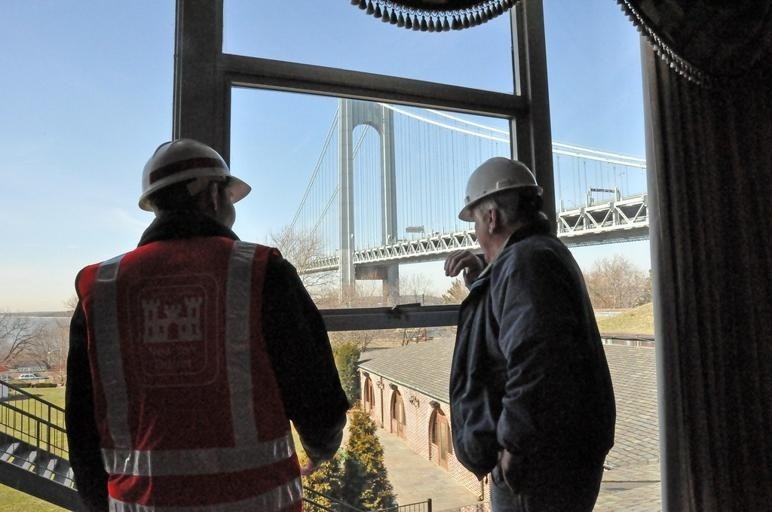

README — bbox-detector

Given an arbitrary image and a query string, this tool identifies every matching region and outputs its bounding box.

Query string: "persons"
[444,158,616,512]
[65,140,351,512]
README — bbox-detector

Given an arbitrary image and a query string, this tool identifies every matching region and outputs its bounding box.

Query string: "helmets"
[458,155,544,223]
[137,137,252,213]
